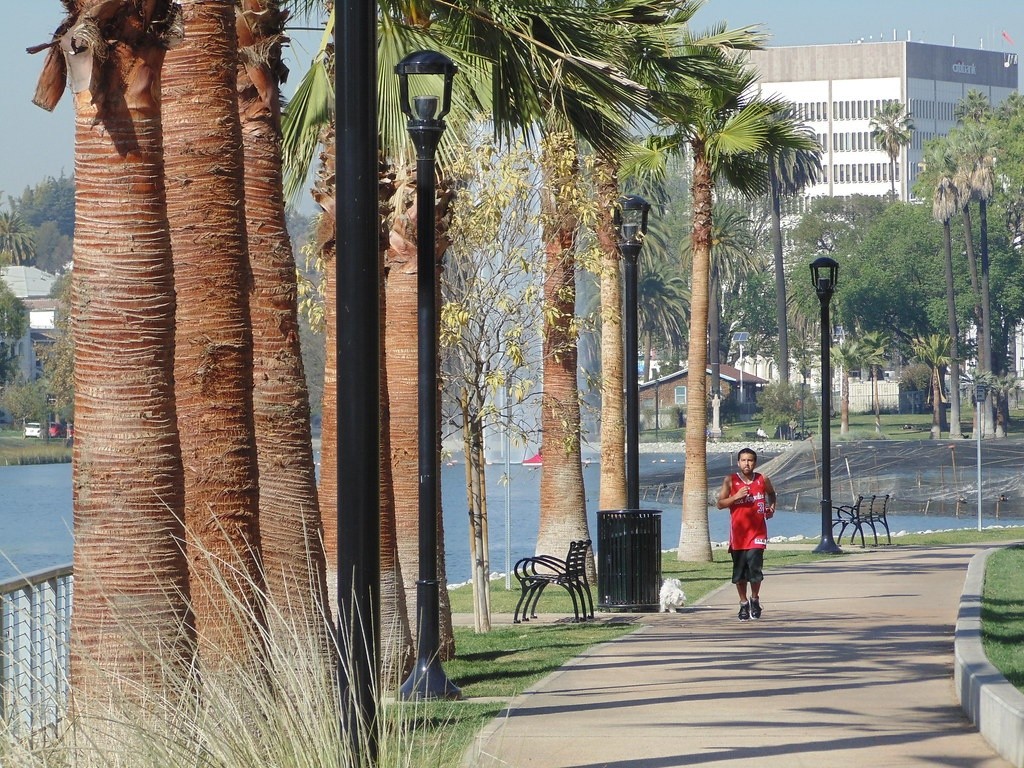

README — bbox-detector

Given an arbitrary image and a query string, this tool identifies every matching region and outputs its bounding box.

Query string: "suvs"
[25,423,41,439]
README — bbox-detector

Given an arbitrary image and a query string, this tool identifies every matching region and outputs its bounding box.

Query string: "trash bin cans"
[597,509,663,611]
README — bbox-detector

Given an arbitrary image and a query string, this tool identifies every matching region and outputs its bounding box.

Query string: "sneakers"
[737,598,750,622]
[750,596,764,619]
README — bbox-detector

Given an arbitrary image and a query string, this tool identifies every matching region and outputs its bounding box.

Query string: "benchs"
[746,432,769,442]
[514,539,594,624]
[831,495,891,548]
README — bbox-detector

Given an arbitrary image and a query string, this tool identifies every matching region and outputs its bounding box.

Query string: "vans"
[49,423,61,439]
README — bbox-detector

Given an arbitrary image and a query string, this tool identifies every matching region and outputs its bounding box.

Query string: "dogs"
[658,577,687,613]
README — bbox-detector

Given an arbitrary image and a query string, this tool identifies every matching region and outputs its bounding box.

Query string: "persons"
[717,448,776,621]
[757,427,769,441]
[789,418,798,440]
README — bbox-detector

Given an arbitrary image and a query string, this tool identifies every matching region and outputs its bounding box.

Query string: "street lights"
[808,258,844,555]
[396,48,461,705]
[610,194,653,512]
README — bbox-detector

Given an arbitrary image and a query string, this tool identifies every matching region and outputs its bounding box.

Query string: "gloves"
[765,507,774,520]
